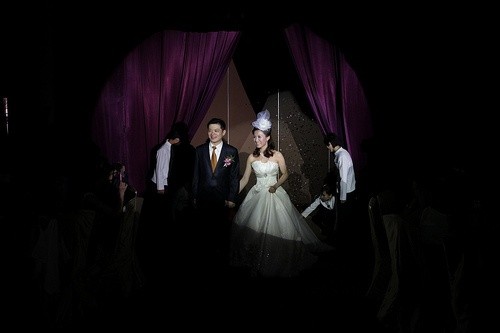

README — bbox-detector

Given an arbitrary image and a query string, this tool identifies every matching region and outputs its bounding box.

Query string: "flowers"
[223,152,235,167]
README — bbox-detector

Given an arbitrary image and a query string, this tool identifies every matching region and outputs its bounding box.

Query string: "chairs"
[368,191,471,333]
[41,196,144,333]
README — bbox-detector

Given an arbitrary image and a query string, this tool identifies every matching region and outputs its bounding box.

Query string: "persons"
[70,161,128,333]
[191,118,238,271]
[300,182,335,237]
[144,121,188,239]
[326,132,358,238]
[229,110,323,278]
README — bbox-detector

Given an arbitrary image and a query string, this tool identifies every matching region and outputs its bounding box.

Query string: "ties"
[211,146,218,172]
